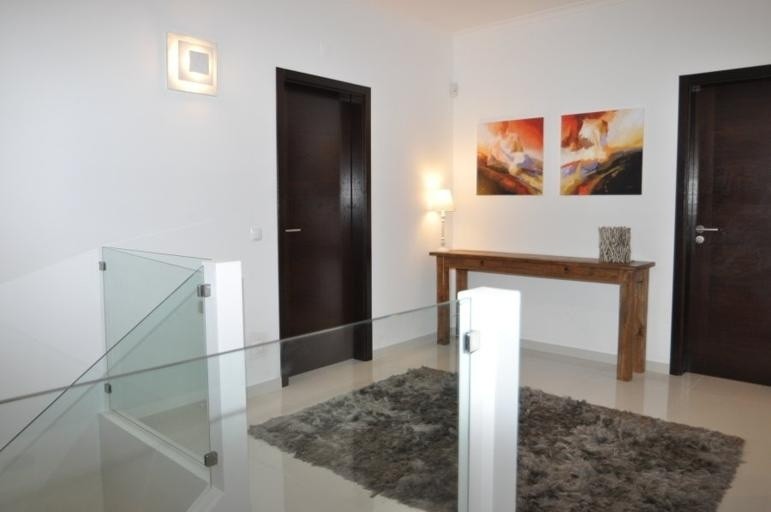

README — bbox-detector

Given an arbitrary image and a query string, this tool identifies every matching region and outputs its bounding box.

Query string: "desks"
[430,247,655,381]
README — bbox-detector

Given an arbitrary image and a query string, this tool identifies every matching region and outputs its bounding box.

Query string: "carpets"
[248,367,745,512]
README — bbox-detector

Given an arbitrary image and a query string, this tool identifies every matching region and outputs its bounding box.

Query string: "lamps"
[166,32,217,96]
[429,189,456,251]
